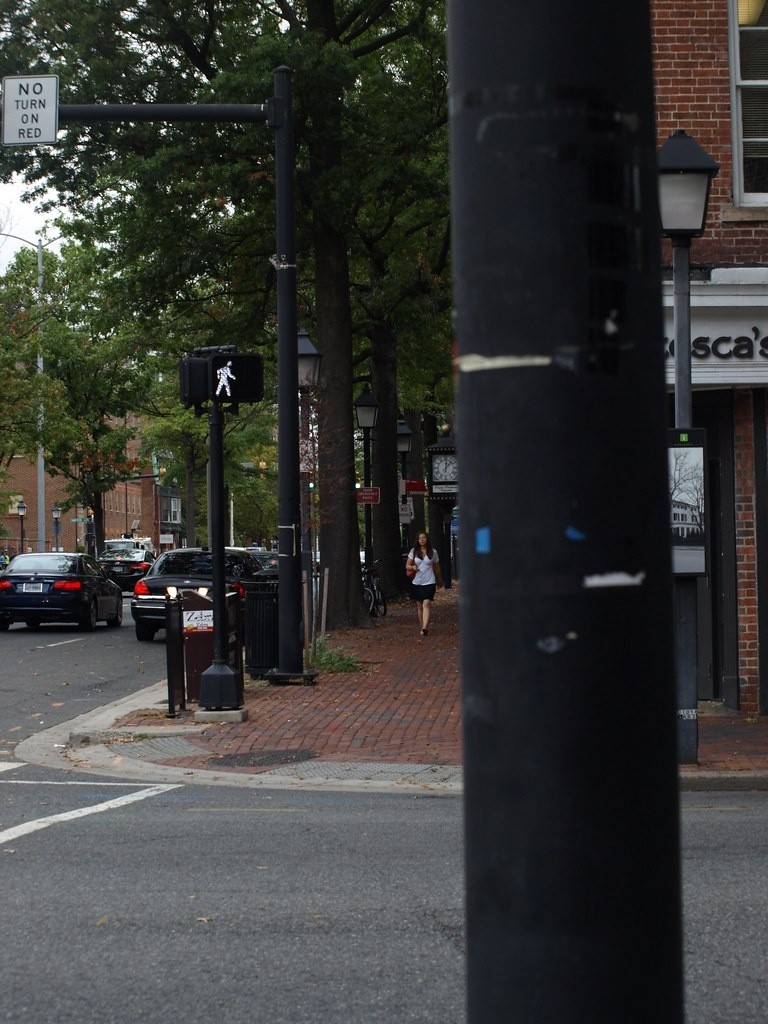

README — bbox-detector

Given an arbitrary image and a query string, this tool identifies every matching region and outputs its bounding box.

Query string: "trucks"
[104,537,153,555]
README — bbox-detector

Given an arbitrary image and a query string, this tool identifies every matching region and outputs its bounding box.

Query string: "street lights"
[51,506,62,552]
[398,421,413,555]
[656,129,718,767]
[353,382,382,615]
[17,501,27,554]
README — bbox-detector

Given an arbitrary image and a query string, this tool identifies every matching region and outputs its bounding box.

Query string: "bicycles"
[360,559,386,617]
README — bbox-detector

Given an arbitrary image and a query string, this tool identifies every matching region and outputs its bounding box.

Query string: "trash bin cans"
[182,587,245,703]
[240,575,306,682]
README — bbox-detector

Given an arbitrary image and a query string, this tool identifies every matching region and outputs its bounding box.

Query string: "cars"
[249,551,320,582]
[0,552,124,633]
[132,545,268,642]
[97,549,157,592]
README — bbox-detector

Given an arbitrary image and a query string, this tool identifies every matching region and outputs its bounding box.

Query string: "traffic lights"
[206,352,265,403]
[179,355,209,410]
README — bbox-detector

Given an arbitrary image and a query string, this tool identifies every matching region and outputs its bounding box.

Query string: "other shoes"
[420,629,428,635]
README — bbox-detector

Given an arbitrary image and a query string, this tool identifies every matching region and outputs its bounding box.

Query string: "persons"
[0,550,10,570]
[405,533,445,637]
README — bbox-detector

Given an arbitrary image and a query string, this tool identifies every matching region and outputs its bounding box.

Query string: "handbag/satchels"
[407,547,417,578]
